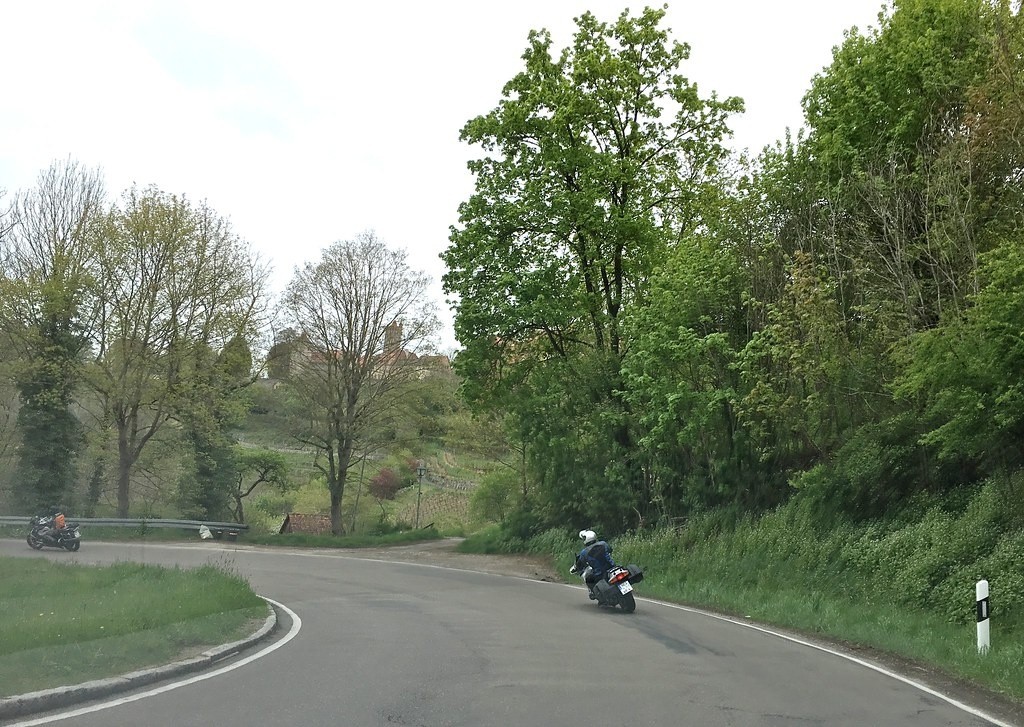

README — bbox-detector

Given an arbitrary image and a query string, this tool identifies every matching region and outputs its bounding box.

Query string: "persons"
[574,530,615,600]
[39,506,65,546]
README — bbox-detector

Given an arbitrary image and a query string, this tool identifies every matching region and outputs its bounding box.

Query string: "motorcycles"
[26,512,82,551]
[569,551,644,614]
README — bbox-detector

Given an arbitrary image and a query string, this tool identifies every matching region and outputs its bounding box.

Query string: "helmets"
[579,529,597,546]
